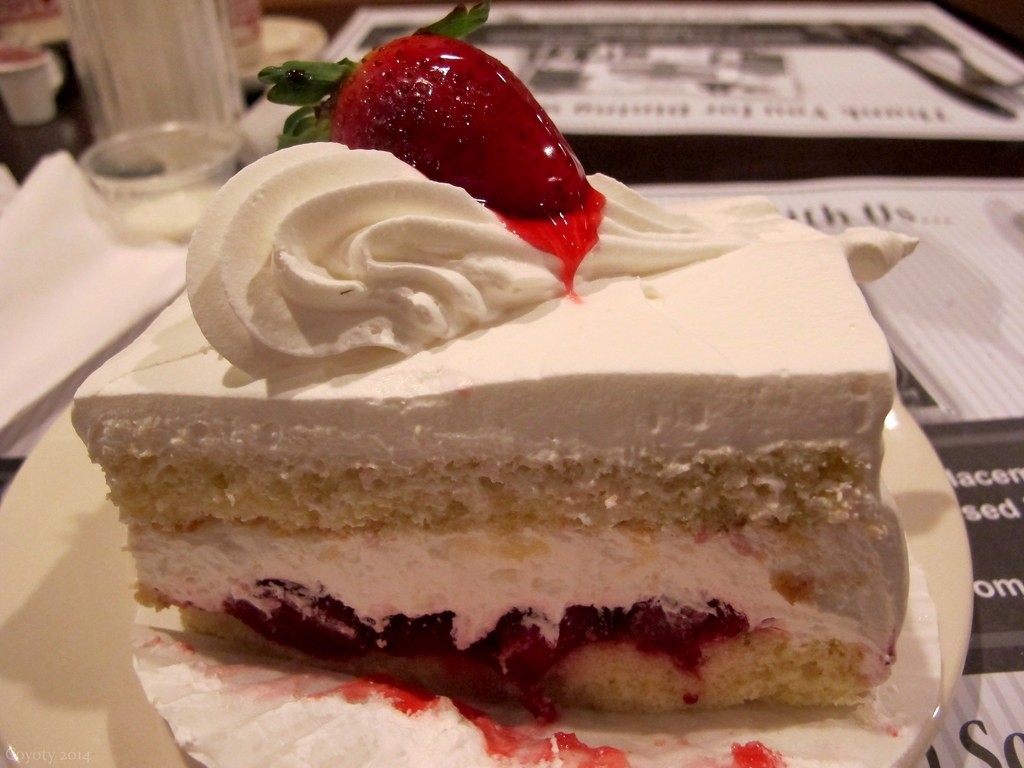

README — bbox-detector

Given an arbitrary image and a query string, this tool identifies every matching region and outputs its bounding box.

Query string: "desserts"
[65,1,922,724]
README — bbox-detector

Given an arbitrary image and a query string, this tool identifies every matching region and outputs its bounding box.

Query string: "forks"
[915,21,1024,117]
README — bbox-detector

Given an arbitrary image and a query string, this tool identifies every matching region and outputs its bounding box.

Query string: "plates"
[236,17,328,91]
[0,379,976,768]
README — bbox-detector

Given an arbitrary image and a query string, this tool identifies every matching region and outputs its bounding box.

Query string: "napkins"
[0,148,194,459]
[858,194,1024,422]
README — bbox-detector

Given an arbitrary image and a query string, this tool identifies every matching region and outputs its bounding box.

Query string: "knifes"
[849,22,1015,118]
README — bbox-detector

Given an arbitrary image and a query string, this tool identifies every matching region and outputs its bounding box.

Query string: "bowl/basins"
[78,121,245,245]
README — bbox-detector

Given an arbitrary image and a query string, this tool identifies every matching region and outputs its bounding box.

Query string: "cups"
[63,0,248,168]
[0,46,60,126]
